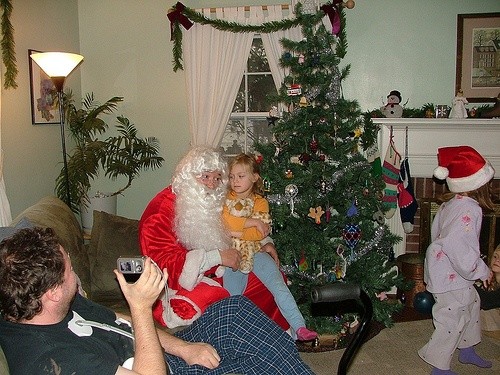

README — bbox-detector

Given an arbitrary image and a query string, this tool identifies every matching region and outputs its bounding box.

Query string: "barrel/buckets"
[395,253,426,309]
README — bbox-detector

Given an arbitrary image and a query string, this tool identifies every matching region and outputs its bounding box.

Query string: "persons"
[138,145,298,343]
[472,242,500,332]
[416,145,496,375]
[0,226,315,375]
[221,153,318,343]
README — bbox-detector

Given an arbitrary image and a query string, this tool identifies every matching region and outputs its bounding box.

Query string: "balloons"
[413,292,434,312]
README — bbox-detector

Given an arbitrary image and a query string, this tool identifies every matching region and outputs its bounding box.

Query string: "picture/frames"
[29,48,65,125]
[455,12,500,103]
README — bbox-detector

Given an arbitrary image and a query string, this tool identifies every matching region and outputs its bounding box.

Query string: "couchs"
[0,198,192,375]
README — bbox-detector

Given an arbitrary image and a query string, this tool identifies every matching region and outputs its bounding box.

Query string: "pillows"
[86,210,140,307]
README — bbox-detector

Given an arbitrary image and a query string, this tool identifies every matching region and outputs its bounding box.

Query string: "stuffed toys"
[449,89,469,118]
[382,89,403,118]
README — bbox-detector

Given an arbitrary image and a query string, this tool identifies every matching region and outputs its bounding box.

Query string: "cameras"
[116,255,145,274]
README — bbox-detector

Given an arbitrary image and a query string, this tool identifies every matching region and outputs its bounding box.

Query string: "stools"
[397,253,426,308]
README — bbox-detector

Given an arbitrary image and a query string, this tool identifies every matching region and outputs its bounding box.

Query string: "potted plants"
[54,87,165,235]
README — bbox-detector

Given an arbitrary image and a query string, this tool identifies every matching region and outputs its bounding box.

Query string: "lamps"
[30,52,84,211]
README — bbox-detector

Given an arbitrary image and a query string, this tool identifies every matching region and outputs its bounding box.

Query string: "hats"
[433,146,495,192]
